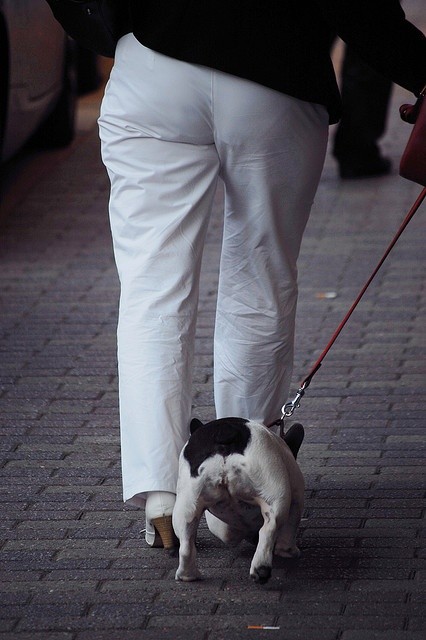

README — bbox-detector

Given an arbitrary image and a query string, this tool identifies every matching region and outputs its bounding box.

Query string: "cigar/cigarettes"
[316,292,337,299]
[247,626,280,630]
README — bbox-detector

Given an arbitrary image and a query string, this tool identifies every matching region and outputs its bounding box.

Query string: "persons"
[95,2,425,555]
[330,43,393,180]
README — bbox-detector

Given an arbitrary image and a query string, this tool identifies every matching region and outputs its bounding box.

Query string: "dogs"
[172,418,305,584]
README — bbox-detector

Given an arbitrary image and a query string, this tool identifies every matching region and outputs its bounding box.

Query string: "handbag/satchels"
[398,87,426,187]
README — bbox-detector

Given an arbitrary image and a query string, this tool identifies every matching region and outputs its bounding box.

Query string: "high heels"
[145,491,176,550]
